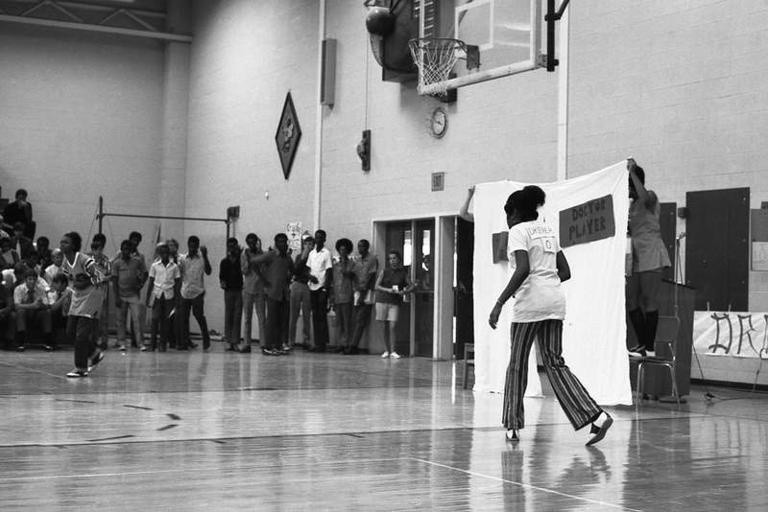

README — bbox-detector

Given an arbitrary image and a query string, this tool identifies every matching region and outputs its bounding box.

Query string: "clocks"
[431,107,448,138]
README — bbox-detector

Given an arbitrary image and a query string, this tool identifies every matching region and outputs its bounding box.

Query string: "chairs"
[629,316,680,409]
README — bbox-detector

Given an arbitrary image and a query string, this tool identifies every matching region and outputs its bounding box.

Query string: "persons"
[1,189,211,376]
[220,229,413,359]
[487,188,611,447]
[627,157,671,359]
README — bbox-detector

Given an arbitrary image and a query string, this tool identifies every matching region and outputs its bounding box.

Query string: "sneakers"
[507,430,519,442]
[382,352,388,357]
[203,342,210,351]
[88,352,104,373]
[263,344,292,356]
[312,344,356,355]
[225,343,251,352]
[629,345,656,357]
[45,345,53,350]
[391,352,400,359]
[67,368,88,377]
[131,340,197,353]
[119,345,127,351]
[585,412,612,446]
[16,346,24,352]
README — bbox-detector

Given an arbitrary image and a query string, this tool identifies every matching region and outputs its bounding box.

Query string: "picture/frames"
[275,92,301,179]
